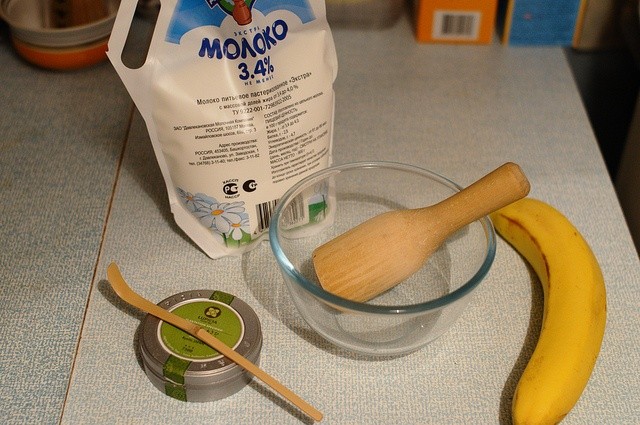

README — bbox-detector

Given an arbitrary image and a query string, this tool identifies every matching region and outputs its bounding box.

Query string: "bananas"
[488,197,608,425]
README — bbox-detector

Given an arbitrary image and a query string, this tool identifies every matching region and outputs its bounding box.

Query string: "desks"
[0,28,640,424]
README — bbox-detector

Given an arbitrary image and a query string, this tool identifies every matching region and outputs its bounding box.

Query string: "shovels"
[312,162,530,312]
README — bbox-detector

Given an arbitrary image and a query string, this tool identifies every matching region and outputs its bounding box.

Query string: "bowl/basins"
[266,160,499,355]
[0,0,121,74]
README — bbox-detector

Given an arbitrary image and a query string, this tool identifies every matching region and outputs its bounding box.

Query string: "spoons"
[107,262,330,422]
